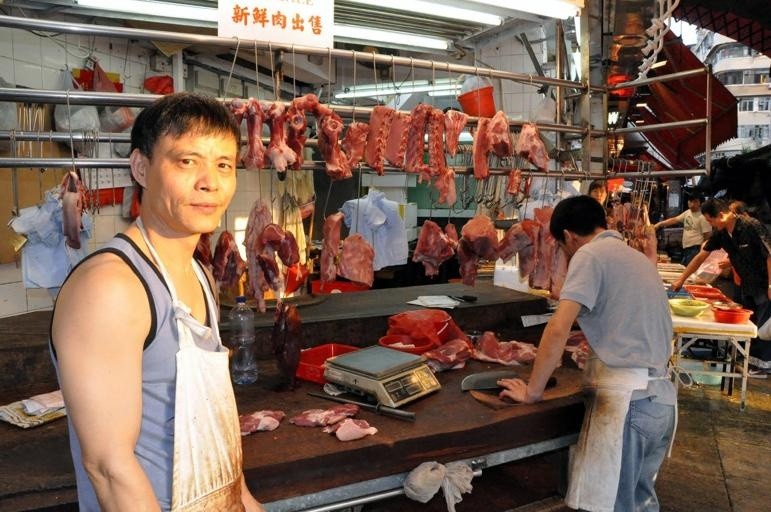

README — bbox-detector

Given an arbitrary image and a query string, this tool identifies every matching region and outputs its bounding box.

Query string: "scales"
[323,345,442,408]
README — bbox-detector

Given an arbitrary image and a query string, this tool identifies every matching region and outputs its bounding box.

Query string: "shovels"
[520,314,554,327]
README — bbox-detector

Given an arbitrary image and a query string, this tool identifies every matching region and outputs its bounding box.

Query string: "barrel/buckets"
[454,73,497,119]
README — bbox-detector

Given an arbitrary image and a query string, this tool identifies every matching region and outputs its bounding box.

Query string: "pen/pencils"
[448,295,465,302]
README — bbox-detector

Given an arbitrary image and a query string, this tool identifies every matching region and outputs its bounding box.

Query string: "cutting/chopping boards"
[470,364,582,410]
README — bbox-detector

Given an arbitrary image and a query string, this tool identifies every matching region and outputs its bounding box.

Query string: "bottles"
[228,296,258,385]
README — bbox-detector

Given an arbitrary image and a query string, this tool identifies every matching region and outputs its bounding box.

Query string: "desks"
[665,281,759,415]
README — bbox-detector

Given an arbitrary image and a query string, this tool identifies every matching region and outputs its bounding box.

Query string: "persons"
[587,179,607,204]
[496,197,678,512]
[48,93,269,512]
[651,192,771,379]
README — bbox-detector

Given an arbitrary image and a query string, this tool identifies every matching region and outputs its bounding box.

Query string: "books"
[419,293,460,307]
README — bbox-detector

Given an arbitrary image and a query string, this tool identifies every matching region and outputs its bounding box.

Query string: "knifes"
[460,369,558,391]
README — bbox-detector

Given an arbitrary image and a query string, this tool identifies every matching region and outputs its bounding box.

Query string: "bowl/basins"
[668,298,710,317]
[711,307,754,324]
[688,361,736,387]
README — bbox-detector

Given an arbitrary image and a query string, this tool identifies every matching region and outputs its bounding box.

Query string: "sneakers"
[737,364,768,379]
